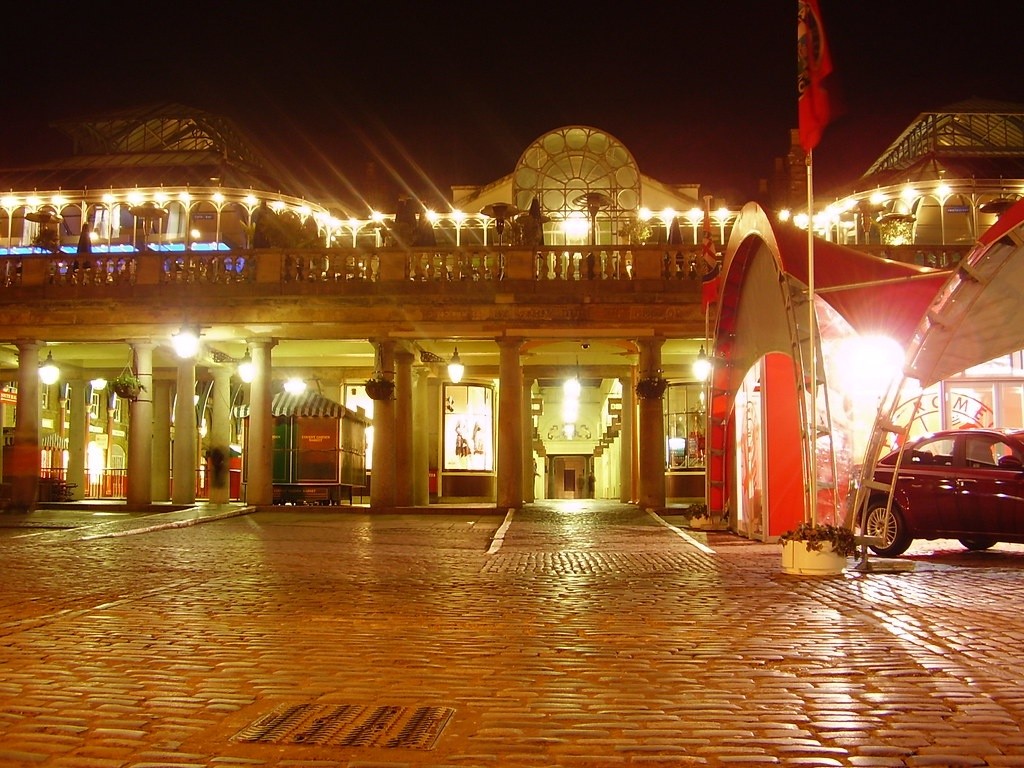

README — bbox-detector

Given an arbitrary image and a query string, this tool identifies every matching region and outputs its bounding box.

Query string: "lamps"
[238,347,257,383]
[37,350,60,385]
[447,346,464,385]
[90,377,108,391]
[693,342,712,381]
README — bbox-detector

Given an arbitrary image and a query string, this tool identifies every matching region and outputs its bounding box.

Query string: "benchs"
[910,451,955,466]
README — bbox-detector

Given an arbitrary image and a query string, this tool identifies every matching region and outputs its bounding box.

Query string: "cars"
[853,429,1024,557]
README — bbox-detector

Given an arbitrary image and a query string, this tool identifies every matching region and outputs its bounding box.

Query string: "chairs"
[969,439,995,468]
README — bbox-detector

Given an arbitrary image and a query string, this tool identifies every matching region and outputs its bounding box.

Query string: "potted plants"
[635,376,670,399]
[111,375,148,398]
[779,519,856,575]
[683,503,728,530]
[364,371,396,400]
